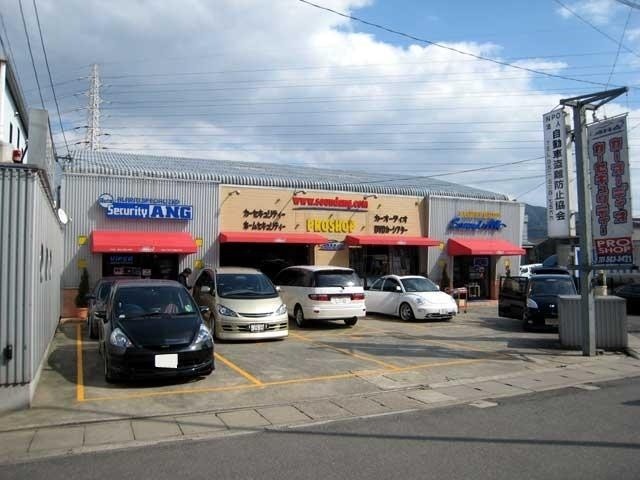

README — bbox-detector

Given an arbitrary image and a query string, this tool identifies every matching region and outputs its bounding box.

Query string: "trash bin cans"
[557,295,627,350]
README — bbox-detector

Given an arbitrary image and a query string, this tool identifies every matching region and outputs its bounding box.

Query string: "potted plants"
[75,268,89,320]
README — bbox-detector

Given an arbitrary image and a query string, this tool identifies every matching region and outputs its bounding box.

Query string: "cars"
[193,267,289,340]
[272,265,367,328]
[498,254,578,327]
[85,275,214,384]
[365,274,458,322]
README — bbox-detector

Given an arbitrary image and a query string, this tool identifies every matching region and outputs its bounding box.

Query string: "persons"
[176,267,193,291]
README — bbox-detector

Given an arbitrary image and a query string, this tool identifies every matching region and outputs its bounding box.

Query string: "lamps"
[294,190,307,195]
[229,190,240,195]
[364,194,378,199]
